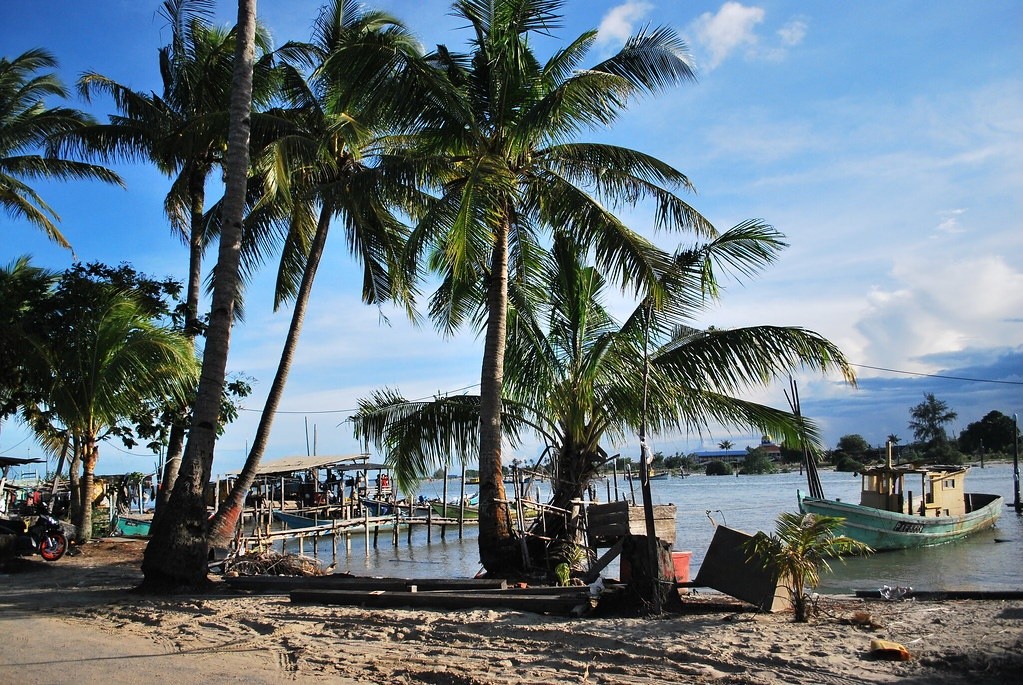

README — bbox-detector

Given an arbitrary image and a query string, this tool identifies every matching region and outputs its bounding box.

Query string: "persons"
[373,474,388,501]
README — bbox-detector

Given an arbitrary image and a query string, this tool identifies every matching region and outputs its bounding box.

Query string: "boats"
[781,375,1005,558]
[94,451,553,546]
[624,468,672,481]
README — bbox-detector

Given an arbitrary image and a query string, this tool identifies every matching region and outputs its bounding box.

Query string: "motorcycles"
[0,499,69,561]
